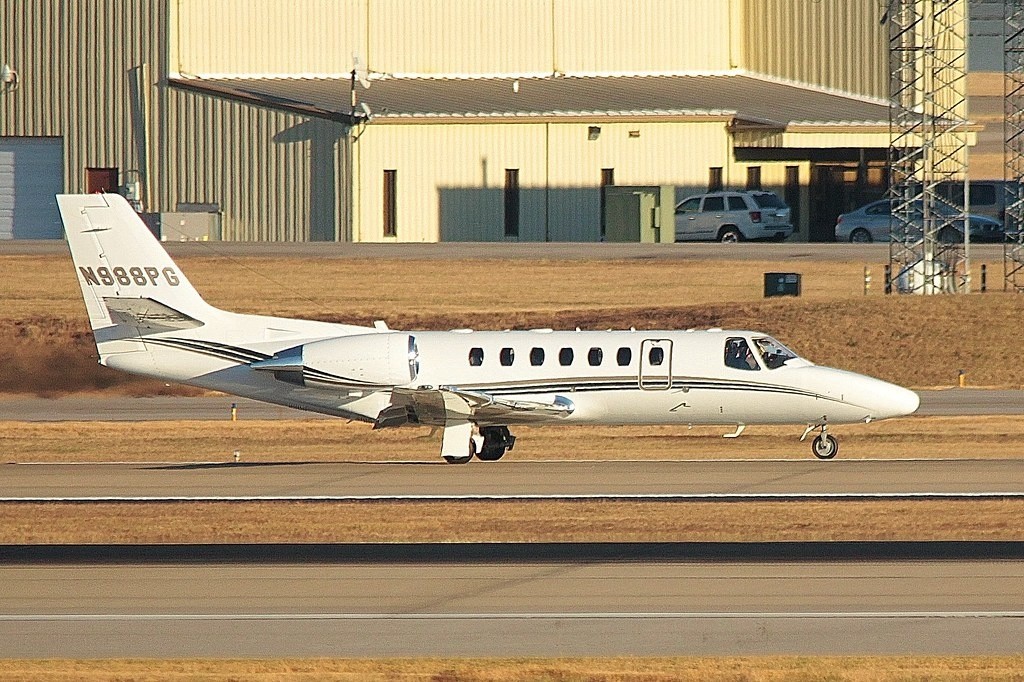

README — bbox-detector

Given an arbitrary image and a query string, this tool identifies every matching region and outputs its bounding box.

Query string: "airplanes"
[56,193,921,464]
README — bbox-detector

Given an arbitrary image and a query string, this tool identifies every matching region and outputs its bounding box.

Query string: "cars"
[835,198,1005,245]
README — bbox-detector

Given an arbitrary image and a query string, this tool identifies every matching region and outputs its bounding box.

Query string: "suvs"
[674,190,792,243]
